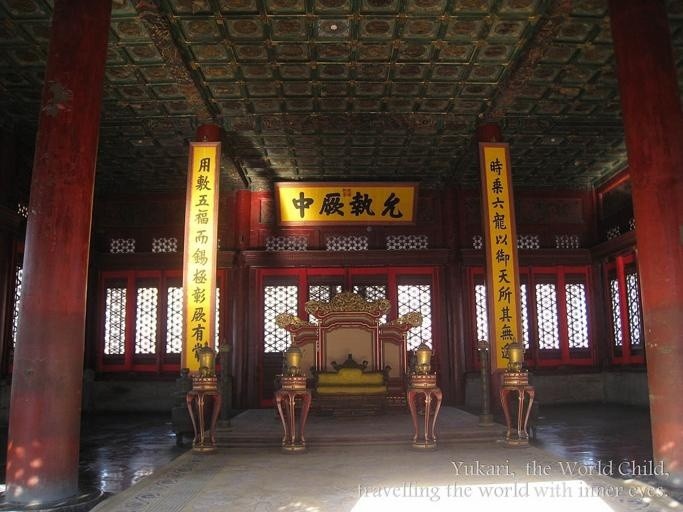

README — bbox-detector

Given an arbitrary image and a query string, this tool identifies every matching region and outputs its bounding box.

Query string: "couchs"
[313,353,393,397]
